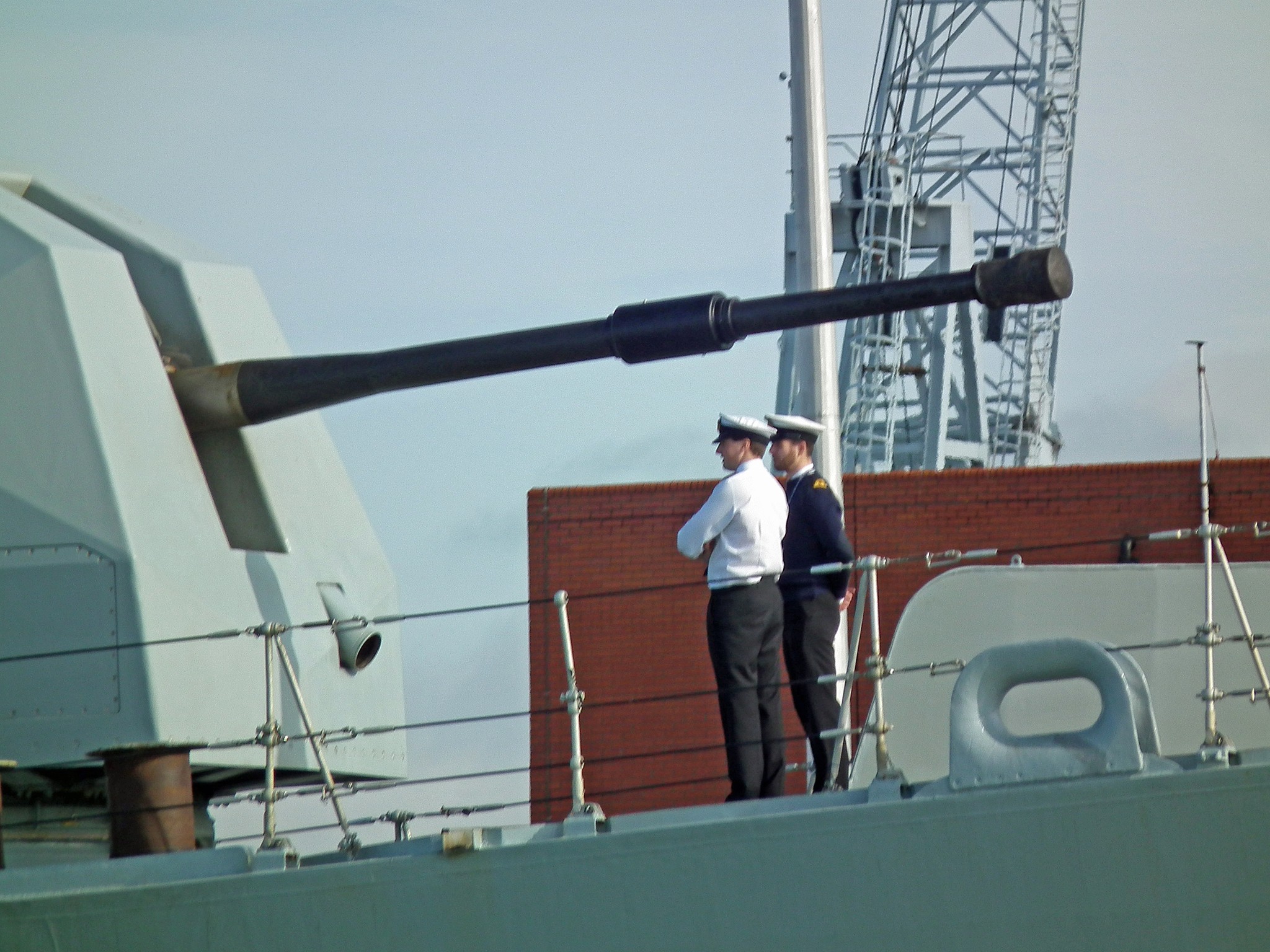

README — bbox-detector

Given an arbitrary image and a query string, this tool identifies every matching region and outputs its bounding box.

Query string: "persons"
[764,413,856,794]
[677,411,788,803]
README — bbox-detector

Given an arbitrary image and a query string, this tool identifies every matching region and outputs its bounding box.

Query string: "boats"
[3,0,1269,952]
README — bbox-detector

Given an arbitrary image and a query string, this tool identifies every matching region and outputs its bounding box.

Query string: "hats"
[712,412,777,443]
[764,415,826,443]
